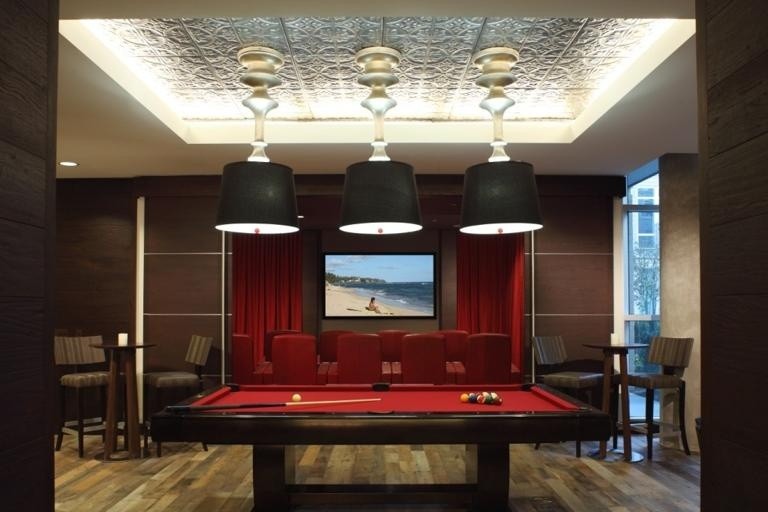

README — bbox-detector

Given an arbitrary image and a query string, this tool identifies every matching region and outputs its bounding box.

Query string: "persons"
[368,297,381,313]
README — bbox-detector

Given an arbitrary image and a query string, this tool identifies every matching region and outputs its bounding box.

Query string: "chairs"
[52,334,126,457]
[231,328,520,385]
[531,335,604,458]
[611,336,694,460]
[143,334,215,456]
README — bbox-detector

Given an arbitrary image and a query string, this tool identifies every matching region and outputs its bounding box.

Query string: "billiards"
[291,394,302,402]
[462,392,503,405]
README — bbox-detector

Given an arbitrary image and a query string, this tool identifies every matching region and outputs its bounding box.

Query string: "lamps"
[214,44,301,237]
[459,46,545,237]
[338,45,424,237]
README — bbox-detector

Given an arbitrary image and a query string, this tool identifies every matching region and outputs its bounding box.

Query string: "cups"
[118,333,129,347]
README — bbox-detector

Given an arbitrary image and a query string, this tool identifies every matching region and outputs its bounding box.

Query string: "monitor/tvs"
[321,251,438,321]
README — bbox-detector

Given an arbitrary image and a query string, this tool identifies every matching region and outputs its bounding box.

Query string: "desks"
[88,342,158,462]
[150,382,613,512]
[580,342,650,464]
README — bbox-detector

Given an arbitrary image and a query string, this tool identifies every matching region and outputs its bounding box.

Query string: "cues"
[166,398,382,410]
